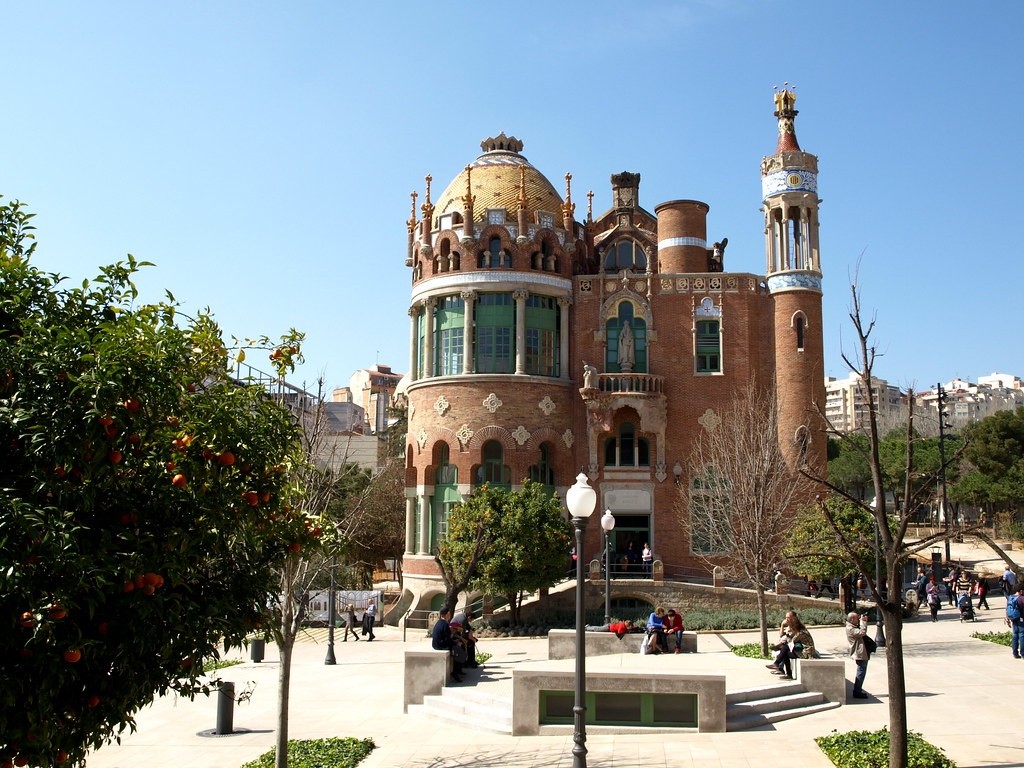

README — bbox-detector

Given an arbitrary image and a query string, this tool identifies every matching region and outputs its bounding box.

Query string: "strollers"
[956,592,976,623]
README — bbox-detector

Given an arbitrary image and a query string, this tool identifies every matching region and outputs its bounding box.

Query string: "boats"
[320,521,342,666]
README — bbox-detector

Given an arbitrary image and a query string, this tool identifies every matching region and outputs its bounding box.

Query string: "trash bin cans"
[251,638,264,663]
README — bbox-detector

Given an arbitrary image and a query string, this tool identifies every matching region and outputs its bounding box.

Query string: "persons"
[926,576,941,622]
[603,545,617,580]
[568,541,576,580]
[945,566,990,610]
[1006,584,1024,658]
[617,320,635,364]
[450,610,480,668]
[342,604,360,642]
[583,365,598,388]
[646,608,684,654]
[642,543,652,578]
[846,612,868,699]
[364,599,376,642]
[625,542,633,576]
[585,620,641,634]
[914,567,928,607]
[1004,566,1016,598]
[766,611,821,679]
[802,569,836,601]
[432,607,466,682]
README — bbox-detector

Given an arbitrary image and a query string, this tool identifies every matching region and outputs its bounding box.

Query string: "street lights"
[566,468,597,768]
[601,507,615,627]
[869,496,886,648]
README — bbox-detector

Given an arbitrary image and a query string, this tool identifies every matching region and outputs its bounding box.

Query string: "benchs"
[772,646,845,704]
[513,660,726,735]
[403,643,459,714]
[548,629,697,662]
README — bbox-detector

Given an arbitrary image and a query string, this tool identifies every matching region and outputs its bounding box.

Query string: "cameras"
[862,612,868,617]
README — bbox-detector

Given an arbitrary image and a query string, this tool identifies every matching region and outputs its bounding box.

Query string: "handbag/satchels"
[640,635,652,654]
[863,635,877,653]
[978,587,984,596]
[928,594,936,604]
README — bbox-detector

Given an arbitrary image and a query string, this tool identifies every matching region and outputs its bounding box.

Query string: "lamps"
[670,458,684,487]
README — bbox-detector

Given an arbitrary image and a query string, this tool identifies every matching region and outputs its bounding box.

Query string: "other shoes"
[976,606,980,609]
[766,663,778,669]
[780,674,793,680]
[772,670,785,674]
[342,639,348,641]
[1013,654,1022,659]
[675,648,679,653]
[661,648,667,653]
[355,637,359,640]
[367,634,375,642]
[451,671,463,683]
[853,692,868,698]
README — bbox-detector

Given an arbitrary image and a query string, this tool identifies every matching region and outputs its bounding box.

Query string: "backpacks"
[1007,595,1020,620]
